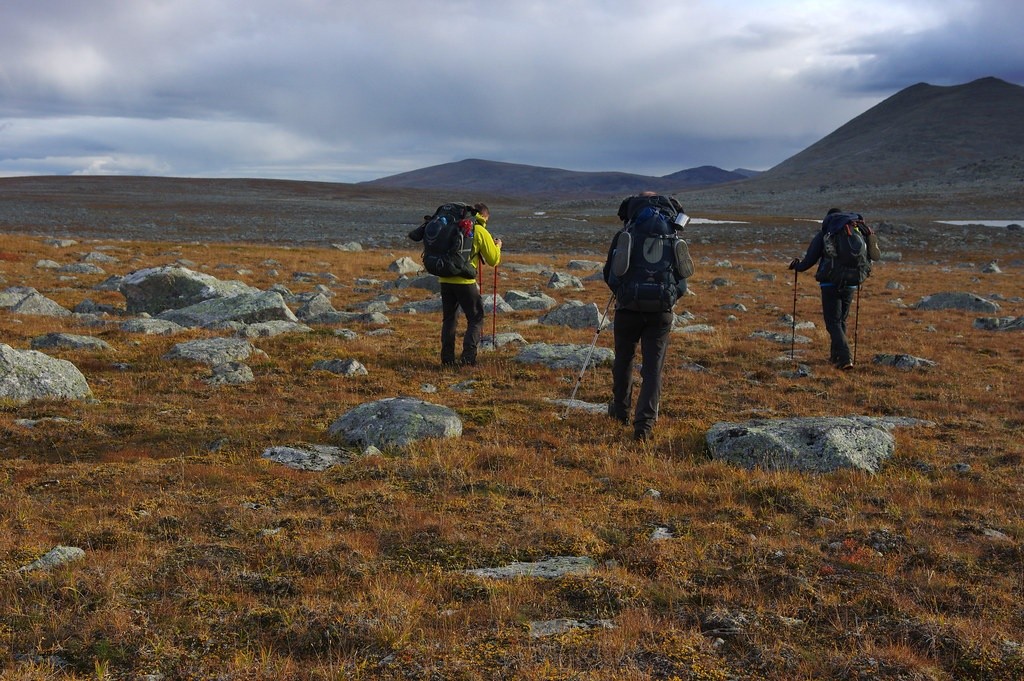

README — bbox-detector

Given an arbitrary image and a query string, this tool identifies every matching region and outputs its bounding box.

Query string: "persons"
[789,208,856,370]
[437,202,502,372]
[602,192,687,444]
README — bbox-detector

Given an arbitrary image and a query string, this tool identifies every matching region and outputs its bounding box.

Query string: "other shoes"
[441,361,459,371]
[867,235,881,260]
[634,428,646,441]
[824,234,835,255]
[829,357,837,365]
[675,239,696,278]
[608,406,630,426]
[611,232,630,276]
[837,358,853,370]
[462,357,480,367]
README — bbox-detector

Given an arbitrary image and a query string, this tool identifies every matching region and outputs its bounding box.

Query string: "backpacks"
[827,219,872,284]
[422,203,478,278]
[609,194,691,281]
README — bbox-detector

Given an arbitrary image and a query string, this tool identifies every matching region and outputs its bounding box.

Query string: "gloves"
[789,259,801,270]
